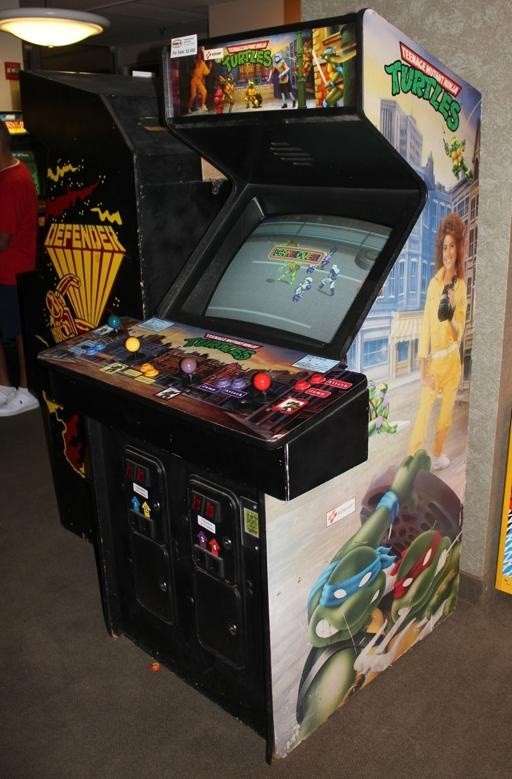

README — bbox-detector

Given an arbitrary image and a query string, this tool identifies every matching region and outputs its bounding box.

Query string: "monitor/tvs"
[204,213,393,344]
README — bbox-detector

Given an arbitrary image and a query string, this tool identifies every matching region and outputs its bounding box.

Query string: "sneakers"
[430,453,450,470]
[0,385,40,417]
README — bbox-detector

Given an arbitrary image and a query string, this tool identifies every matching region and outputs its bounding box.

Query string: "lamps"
[1,8,110,50]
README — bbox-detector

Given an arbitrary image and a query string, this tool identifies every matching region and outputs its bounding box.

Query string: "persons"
[0,117,49,418]
[406,211,469,476]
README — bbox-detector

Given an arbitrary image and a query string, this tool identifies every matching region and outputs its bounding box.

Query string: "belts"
[432,344,457,360]
